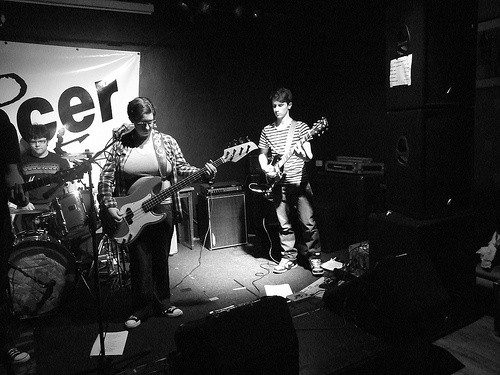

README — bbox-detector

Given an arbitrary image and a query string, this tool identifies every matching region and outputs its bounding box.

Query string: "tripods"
[100,246,132,285]
[43,138,152,375]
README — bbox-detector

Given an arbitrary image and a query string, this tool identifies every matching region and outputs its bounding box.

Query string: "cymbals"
[9,207,42,214]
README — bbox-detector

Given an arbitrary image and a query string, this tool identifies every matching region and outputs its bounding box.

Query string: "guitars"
[101,135,258,246]
[0,164,85,205]
[259,116,329,208]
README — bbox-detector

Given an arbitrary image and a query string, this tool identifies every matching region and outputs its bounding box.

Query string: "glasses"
[136,119,156,127]
[30,139,48,145]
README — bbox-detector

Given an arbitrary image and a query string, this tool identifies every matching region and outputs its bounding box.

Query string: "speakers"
[383,112,473,216]
[310,171,371,251]
[326,251,416,335]
[365,210,477,342]
[172,295,300,375]
[196,191,249,250]
[383,0,476,111]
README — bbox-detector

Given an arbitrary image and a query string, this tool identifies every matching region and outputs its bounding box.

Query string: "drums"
[53,190,88,233]
[5,229,80,321]
[36,210,67,240]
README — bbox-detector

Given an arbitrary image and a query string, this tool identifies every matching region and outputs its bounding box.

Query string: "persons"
[258,88,324,276]
[97,97,217,327]
[0,110,30,362]
[13,125,92,239]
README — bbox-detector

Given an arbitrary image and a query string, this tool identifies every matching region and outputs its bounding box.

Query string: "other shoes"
[273,258,298,273]
[309,258,325,274]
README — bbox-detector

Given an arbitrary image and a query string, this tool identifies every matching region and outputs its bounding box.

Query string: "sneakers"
[2,348,30,364]
[159,305,183,317]
[124,310,146,329]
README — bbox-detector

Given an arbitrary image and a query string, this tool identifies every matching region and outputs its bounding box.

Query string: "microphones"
[47,280,56,287]
[114,122,131,138]
